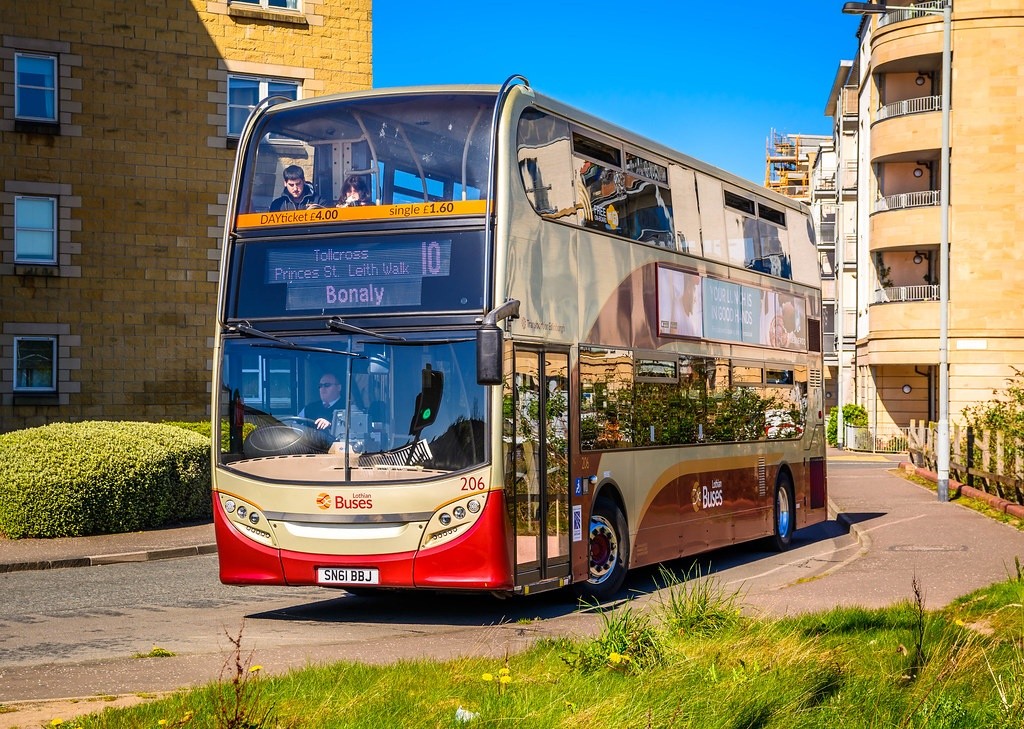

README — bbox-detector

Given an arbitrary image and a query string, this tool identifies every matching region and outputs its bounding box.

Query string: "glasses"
[317,383,337,388]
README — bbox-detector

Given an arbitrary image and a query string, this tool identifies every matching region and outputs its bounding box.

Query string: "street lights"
[841,1,954,503]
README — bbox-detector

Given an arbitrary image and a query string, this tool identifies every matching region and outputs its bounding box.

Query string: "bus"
[209,73,831,610]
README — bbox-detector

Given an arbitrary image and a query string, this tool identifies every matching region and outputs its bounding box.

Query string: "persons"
[334,174,377,208]
[270,164,334,212]
[293,371,365,430]
